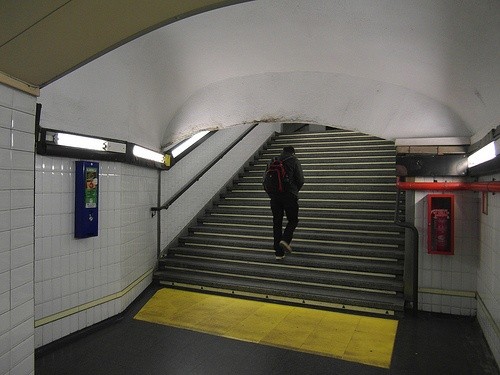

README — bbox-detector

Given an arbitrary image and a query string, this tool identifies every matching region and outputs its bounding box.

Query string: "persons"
[264,146,305,259]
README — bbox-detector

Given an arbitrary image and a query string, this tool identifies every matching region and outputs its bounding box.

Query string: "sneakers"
[279,240,291,254]
[276,255,286,259]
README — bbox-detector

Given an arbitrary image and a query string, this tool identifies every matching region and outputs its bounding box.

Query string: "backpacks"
[262,156,295,197]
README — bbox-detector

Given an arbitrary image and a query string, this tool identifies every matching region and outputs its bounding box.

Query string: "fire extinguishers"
[435,210,447,250]
